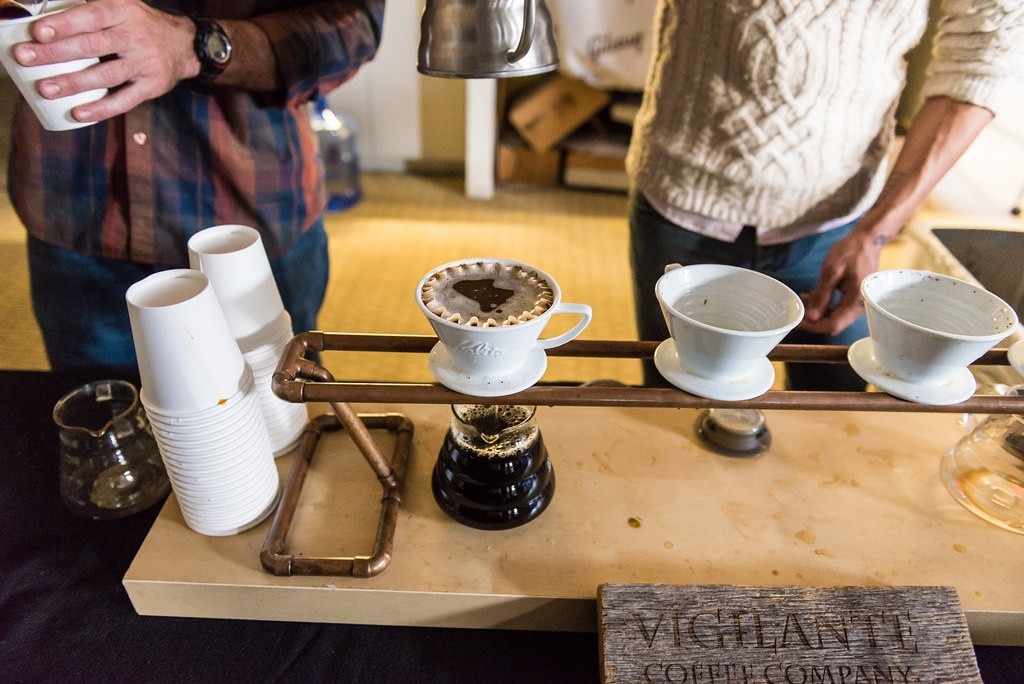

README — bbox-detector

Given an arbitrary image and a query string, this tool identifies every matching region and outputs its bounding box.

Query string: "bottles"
[307,97,361,213]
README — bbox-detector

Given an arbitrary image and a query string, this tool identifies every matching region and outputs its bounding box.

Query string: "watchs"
[183,10,233,92]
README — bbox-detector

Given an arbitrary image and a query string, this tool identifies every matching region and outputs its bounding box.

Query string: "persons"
[0,0,384,377]
[625,0,1024,393]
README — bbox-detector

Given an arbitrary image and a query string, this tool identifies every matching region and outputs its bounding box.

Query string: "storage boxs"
[499,145,560,189]
[510,74,609,152]
[565,142,631,193]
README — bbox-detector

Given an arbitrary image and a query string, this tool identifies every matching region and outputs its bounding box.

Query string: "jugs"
[430,402,555,531]
[51,376,171,520]
[941,382,1024,532]
[417,0,560,79]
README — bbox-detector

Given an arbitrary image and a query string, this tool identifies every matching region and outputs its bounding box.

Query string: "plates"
[1006,340,1024,381]
[848,336,977,406]
[428,338,549,398]
[654,337,775,402]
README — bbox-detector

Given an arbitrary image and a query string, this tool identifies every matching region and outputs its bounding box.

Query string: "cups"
[125,223,310,538]
[656,262,805,381]
[414,256,594,380]
[861,270,1017,384]
[0,0,110,131]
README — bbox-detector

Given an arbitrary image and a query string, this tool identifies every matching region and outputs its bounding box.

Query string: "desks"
[0,368,1024,684]
